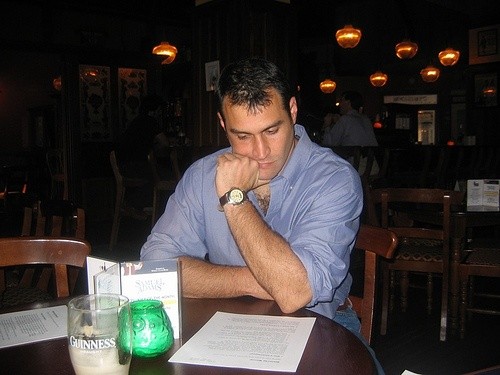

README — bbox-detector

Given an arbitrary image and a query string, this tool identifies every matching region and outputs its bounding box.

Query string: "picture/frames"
[204,58,221,95]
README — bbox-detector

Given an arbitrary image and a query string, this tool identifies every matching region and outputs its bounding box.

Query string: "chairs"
[0,200,92,304]
[170,145,184,178]
[110,150,159,250]
[339,180,500,345]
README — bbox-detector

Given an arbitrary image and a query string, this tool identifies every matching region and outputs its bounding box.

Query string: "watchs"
[219,187,249,212]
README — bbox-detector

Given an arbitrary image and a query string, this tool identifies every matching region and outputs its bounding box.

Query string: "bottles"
[374,112,385,146]
[119,299,173,358]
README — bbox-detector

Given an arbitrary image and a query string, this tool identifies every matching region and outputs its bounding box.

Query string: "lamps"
[152,30,180,65]
[319,14,461,94]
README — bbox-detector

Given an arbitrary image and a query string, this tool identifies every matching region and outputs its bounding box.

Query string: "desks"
[0,296,377,375]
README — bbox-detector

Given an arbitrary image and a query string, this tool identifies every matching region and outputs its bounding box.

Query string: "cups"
[66,293,132,375]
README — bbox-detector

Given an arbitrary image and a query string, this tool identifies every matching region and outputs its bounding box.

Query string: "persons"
[121,95,181,211]
[322,91,379,187]
[139,59,385,375]
